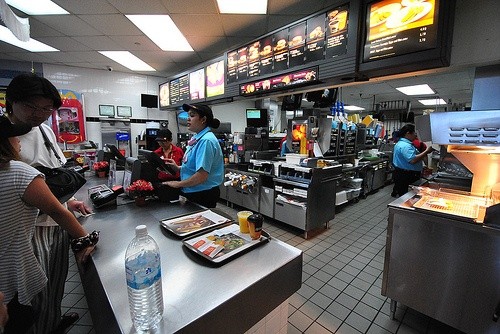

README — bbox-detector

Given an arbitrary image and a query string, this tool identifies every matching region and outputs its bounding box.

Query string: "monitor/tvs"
[106,144,126,161]
[138,149,177,175]
[141,94,158,108]
[246,109,267,127]
[89,140,98,149]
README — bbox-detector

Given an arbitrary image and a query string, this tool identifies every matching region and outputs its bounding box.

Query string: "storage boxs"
[286,154,309,165]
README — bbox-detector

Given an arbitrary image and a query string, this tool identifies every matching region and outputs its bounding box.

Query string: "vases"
[97,171,106,178]
[133,193,145,206]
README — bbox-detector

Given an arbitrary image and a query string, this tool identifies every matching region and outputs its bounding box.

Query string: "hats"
[405,124,415,132]
[154,129,172,141]
[183,103,213,122]
[0,110,32,137]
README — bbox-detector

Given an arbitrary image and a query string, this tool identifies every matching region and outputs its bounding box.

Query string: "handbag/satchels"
[33,125,87,216]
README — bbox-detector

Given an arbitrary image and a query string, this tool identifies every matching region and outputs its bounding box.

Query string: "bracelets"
[71,230,100,252]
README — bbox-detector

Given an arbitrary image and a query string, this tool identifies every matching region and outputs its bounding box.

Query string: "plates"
[370,2,401,28]
[385,2,433,29]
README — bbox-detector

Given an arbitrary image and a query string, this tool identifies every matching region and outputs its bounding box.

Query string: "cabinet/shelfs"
[219,164,342,238]
[320,122,358,164]
[358,129,383,152]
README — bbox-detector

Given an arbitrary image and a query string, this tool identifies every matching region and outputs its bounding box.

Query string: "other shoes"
[59,312,79,332]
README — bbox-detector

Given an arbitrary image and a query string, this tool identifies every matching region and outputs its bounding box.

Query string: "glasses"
[20,100,54,112]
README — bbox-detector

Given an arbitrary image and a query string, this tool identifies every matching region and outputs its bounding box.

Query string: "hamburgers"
[293,36,302,43]
[277,39,286,50]
[264,45,272,54]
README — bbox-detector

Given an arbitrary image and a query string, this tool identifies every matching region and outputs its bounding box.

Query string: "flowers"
[126,179,154,194]
[92,160,108,171]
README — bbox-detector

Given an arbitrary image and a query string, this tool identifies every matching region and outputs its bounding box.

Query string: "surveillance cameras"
[107,67,113,72]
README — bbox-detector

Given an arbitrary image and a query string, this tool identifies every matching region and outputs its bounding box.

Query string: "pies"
[310,26,322,38]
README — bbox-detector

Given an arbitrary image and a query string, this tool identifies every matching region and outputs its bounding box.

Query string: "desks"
[63,160,304,334]
[382,189,500,334]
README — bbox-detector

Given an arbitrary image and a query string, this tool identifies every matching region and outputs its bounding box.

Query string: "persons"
[0,71,93,334]
[162,103,224,208]
[153,129,184,195]
[389,124,433,198]
[0,113,94,334]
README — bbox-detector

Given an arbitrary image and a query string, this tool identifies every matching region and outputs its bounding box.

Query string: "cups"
[246,213,264,240]
[328,9,348,32]
[237,210,254,234]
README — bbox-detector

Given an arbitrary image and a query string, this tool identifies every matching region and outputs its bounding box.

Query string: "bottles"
[124,224,164,330]
